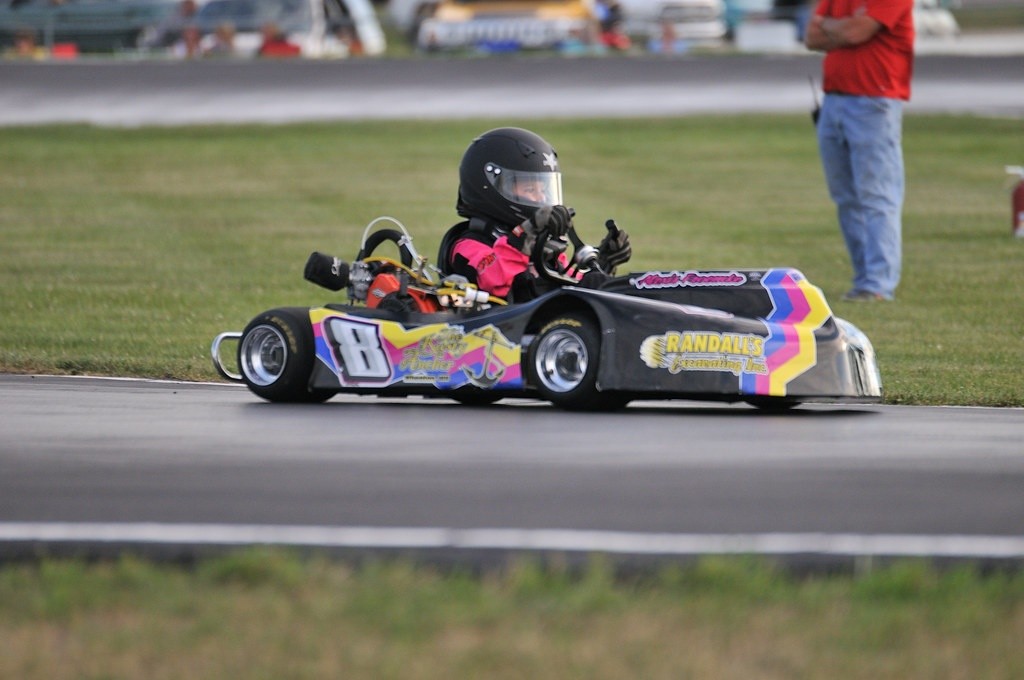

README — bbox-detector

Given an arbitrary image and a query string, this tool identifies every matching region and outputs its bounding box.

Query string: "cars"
[0,0,385,61]
[411,1,809,59]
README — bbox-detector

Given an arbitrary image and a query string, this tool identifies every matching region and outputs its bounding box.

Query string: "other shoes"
[844,286,884,302]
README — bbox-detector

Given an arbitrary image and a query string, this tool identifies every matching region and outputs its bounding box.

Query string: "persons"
[599,5,633,51]
[178,22,371,61]
[2,24,81,61]
[432,126,634,307]
[803,0,915,302]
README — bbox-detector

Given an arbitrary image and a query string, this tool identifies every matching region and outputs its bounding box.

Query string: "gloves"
[521,205,571,240]
[597,230,632,272]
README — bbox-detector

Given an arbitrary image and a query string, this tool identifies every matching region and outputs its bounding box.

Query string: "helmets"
[456,127,563,227]
